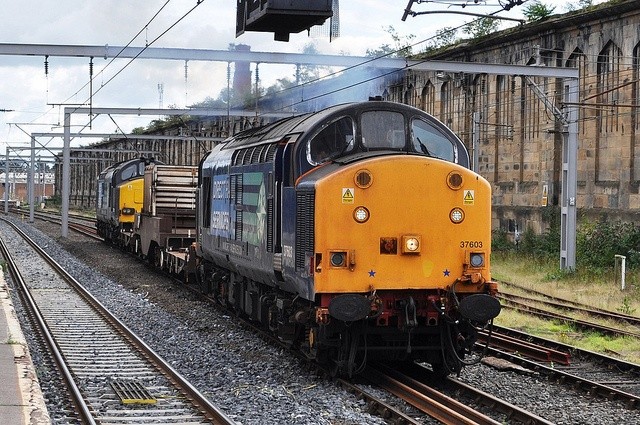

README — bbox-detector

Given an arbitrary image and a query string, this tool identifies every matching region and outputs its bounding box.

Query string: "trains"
[95,96,500,383]
